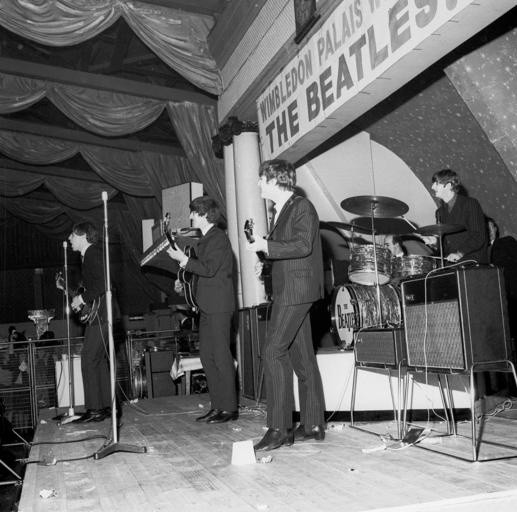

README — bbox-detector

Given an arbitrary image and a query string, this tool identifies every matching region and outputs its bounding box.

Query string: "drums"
[390,255,435,282]
[347,244,393,287]
[328,283,402,350]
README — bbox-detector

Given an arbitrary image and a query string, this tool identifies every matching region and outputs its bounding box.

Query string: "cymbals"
[414,224,466,236]
[341,196,408,219]
[351,217,418,234]
[328,222,378,235]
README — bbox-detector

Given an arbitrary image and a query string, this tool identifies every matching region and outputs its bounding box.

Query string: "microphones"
[101,191,108,227]
[62,241,68,265]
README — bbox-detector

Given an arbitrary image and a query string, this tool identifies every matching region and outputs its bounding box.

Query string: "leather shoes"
[205,409,239,422]
[70,408,109,424]
[195,407,221,422]
[72,405,123,417]
[292,423,325,441]
[253,424,294,451]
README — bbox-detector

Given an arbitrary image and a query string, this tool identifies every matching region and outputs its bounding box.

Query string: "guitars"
[244,219,273,301]
[54,272,100,325]
[163,212,200,315]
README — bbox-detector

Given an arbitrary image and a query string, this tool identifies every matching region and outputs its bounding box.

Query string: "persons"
[68,219,122,424]
[174,309,191,356]
[428,169,490,270]
[241,159,327,453]
[174,195,239,424]
[8,325,58,407]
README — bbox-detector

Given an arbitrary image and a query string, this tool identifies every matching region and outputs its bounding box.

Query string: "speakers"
[399,263,512,374]
[352,328,407,369]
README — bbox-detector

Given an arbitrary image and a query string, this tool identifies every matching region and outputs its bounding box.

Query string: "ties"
[443,202,451,218]
